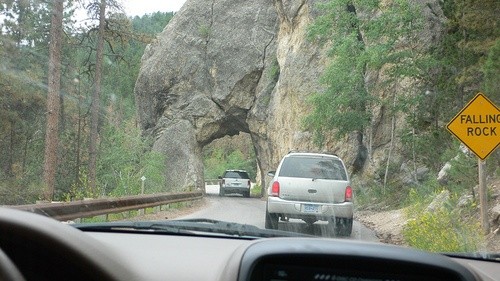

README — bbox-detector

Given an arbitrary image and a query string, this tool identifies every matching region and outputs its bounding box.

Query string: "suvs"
[218,168,252,196]
[265,151,353,236]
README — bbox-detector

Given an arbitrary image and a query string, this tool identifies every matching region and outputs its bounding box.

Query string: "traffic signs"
[447,93,500,158]
